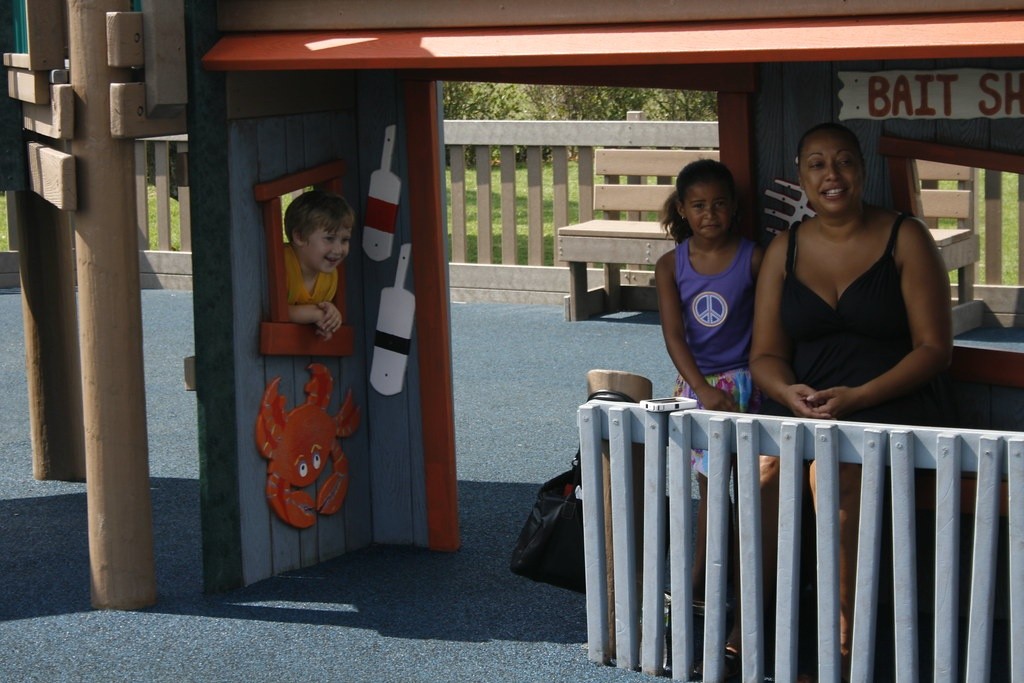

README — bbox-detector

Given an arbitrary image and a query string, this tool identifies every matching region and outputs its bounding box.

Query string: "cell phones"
[639,396,697,411]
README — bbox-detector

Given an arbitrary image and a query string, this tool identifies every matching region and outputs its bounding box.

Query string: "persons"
[654,121,953,683]
[284,190,357,342]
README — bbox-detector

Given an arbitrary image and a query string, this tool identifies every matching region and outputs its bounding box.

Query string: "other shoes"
[693,641,741,679]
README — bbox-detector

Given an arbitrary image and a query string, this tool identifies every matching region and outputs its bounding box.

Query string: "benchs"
[557,148,983,339]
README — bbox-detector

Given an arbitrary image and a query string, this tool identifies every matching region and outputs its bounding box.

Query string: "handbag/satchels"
[510,390,670,595]
